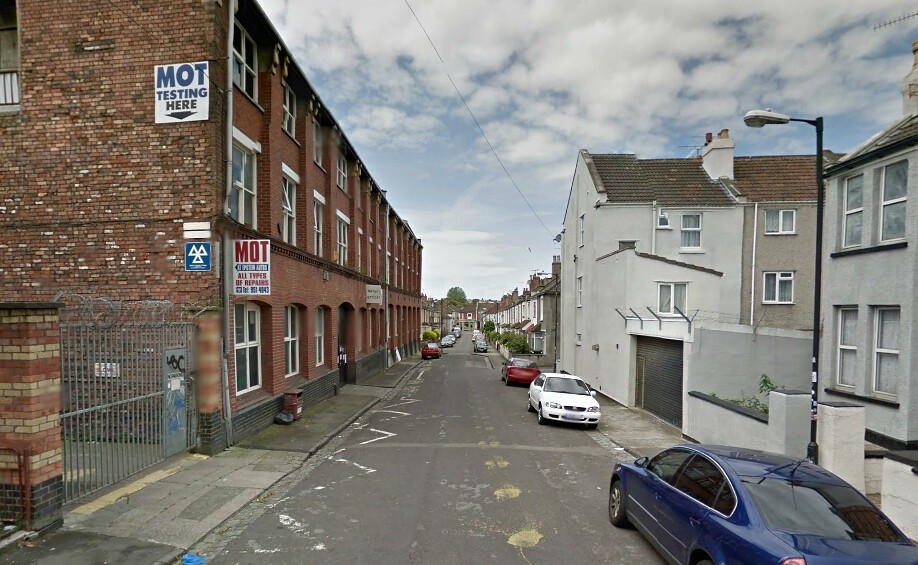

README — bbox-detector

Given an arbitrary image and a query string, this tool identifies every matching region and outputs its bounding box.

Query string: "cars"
[446,326,461,344]
[609,444,918,565]
[471,329,488,353]
[441,337,453,347]
[422,343,442,359]
[501,358,541,386]
[527,373,601,429]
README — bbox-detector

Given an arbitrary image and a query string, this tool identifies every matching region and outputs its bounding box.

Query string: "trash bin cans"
[284,389,304,421]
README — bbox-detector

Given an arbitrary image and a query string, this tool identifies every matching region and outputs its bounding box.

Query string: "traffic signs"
[154,60,210,124]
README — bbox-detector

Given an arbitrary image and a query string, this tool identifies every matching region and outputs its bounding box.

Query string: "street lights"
[743,107,823,465]
[537,272,558,374]
